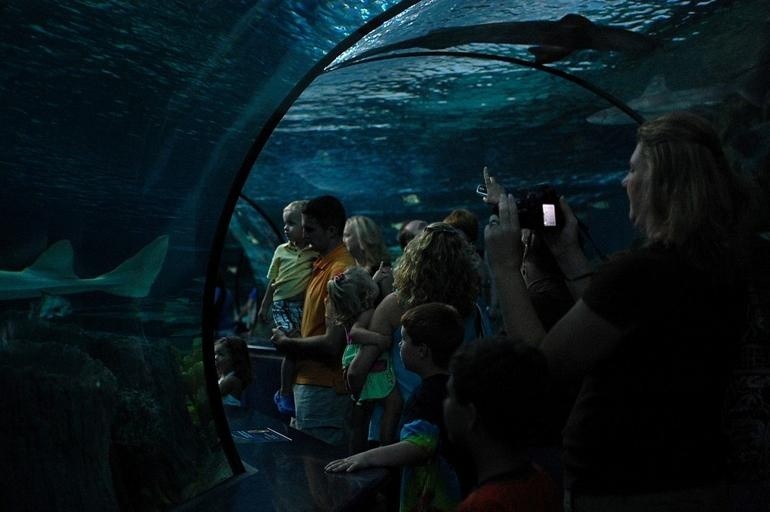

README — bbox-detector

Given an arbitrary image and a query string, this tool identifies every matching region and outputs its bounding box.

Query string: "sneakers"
[272,390,296,416]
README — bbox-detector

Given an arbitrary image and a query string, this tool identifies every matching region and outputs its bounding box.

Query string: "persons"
[213,112,769,512]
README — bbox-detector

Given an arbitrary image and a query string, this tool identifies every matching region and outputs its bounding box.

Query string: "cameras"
[491,184,565,238]
